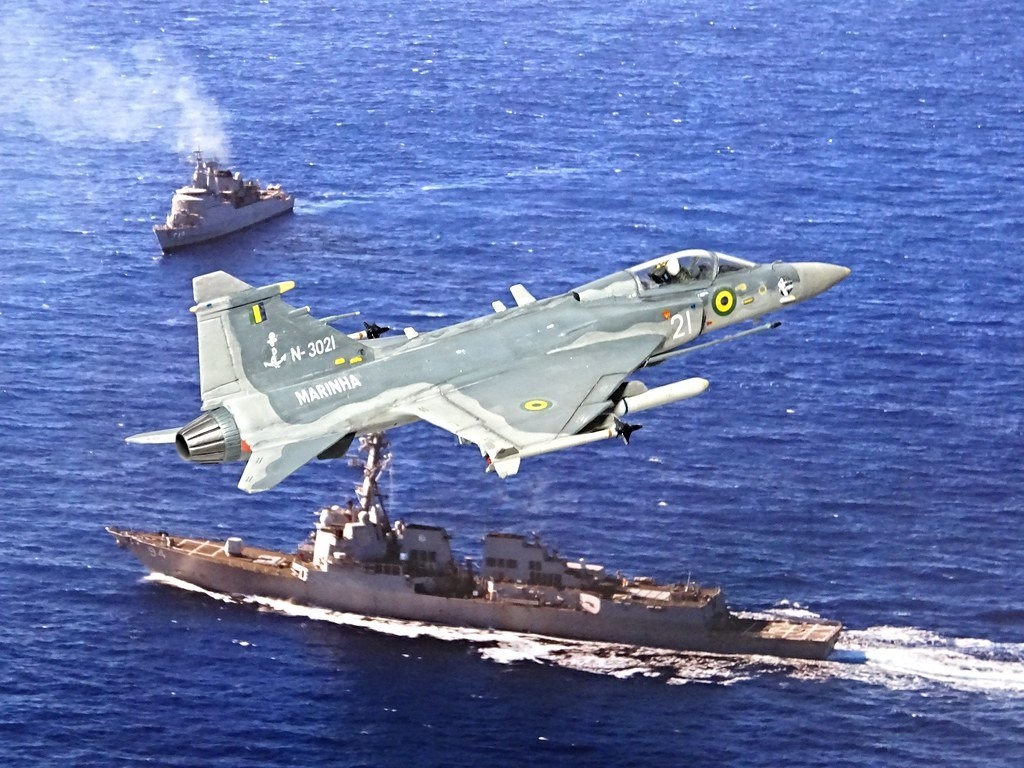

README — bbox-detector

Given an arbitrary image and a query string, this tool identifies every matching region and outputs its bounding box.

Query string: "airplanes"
[124,249,853,494]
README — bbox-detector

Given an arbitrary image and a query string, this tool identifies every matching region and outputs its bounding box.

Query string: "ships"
[104,428,842,660]
[153,144,294,254]
